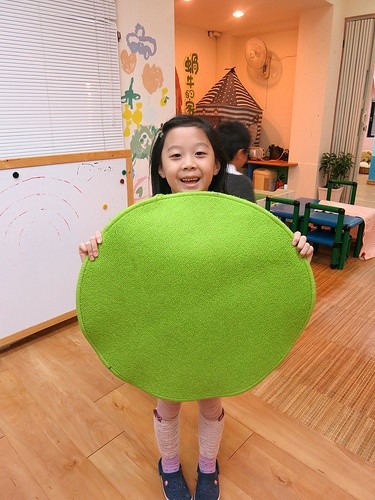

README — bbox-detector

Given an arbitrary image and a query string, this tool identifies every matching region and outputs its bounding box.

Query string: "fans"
[245,38,271,79]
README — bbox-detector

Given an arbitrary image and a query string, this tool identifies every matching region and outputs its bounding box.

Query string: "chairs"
[327,180,358,206]
[266,196,303,234]
[300,202,352,269]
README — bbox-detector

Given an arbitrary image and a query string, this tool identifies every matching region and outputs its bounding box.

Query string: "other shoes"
[194,459,220,500]
[158,456,193,500]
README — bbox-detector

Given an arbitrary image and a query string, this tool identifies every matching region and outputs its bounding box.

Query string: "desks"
[270,197,365,270]
[248,159,298,192]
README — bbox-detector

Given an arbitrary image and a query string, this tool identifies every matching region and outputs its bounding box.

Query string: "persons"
[79,114,314,500]
[213,121,255,203]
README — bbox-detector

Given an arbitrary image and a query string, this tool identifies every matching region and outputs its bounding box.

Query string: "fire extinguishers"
[275,174,285,190]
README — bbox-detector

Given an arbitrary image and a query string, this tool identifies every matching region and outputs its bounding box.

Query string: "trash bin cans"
[253,168,277,192]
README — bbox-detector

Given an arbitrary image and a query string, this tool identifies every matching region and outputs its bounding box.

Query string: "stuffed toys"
[360,151,371,164]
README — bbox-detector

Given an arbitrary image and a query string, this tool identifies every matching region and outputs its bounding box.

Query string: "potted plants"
[317,151,356,202]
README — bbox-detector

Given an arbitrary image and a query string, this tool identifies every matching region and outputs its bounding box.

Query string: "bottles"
[249,147,261,160]
